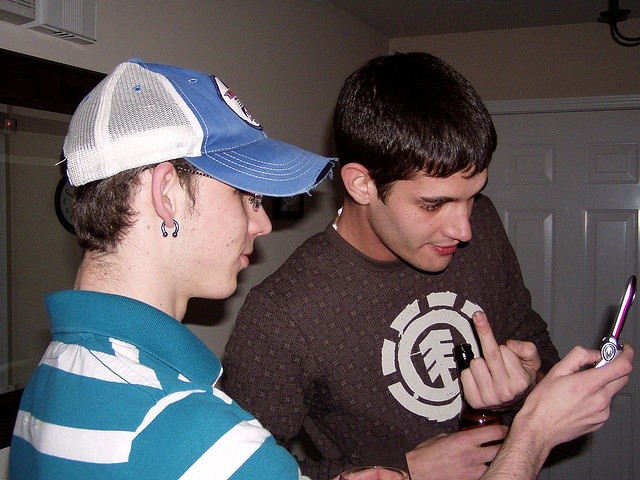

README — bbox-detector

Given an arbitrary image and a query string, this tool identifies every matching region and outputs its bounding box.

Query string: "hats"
[57,59,340,198]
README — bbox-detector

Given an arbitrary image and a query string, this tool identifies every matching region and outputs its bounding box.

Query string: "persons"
[8,56,636,480]
[222,49,560,480]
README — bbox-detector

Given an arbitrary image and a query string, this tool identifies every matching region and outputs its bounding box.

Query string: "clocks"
[55,175,80,236]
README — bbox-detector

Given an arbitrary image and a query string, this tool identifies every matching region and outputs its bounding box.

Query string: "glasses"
[176,167,264,212]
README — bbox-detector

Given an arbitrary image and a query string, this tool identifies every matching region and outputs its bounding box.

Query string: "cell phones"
[590,273,637,370]
